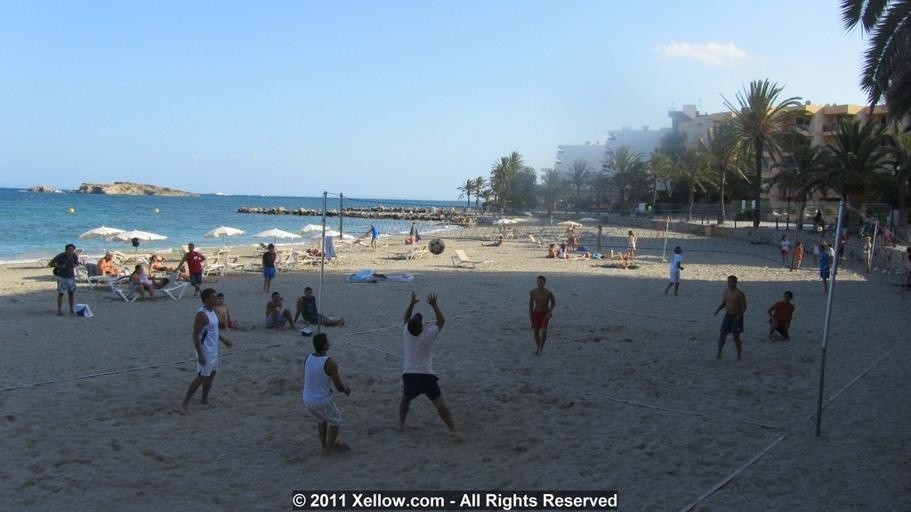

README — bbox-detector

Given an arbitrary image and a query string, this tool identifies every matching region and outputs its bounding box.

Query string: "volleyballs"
[817,226,823,232]
[429,239,445,254]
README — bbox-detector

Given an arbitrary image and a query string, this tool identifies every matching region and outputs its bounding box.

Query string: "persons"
[529,275,556,356]
[766,291,795,340]
[265,293,296,332]
[214,293,254,334]
[544,225,637,268]
[95,244,206,301]
[262,243,276,293]
[481,235,503,247]
[48,243,80,316]
[180,288,233,415]
[779,209,911,295]
[397,289,456,435]
[714,275,748,361]
[293,287,344,328]
[409,222,416,243]
[302,334,351,457]
[306,248,324,256]
[367,223,379,251]
[663,247,685,296]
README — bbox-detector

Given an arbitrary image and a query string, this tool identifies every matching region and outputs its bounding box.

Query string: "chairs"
[388,244,426,261]
[528,233,560,249]
[450,249,492,270]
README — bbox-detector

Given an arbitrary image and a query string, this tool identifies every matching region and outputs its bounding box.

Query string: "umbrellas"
[333,234,356,243]
[298,223,330,237]
[104,229,167,254]
[251,228,299,245]
[577,217,598,223]
[79,226,125,254]
[314,231,341,239]
[557,219,582,227]
[203,226,243,250]
[493,216,541,225]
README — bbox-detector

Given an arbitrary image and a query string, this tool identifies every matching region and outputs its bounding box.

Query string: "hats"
[408,314,422,335]
